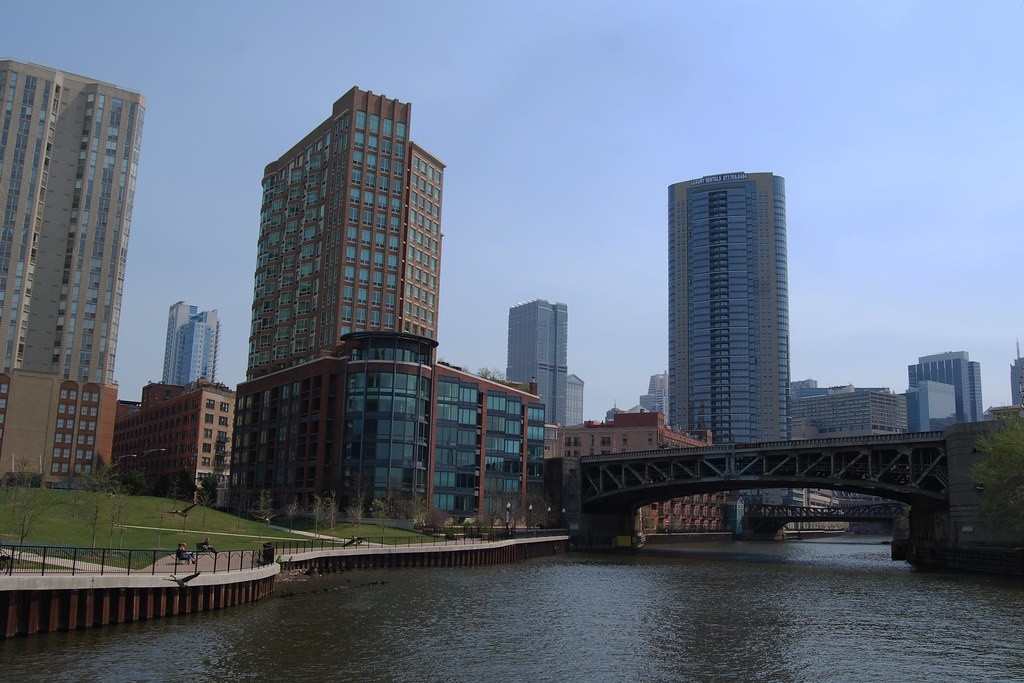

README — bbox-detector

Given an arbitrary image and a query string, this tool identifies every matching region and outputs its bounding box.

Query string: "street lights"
[527,503,533,529]
[138,447,166,474]
[547,506,551,529]
[505,502,512,530]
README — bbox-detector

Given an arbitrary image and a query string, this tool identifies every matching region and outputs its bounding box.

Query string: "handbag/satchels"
[203,545,208,550]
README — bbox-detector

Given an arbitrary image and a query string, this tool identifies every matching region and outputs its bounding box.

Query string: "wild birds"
[162,571,201,589]
[165,502,199,518]
[341,534,364,547]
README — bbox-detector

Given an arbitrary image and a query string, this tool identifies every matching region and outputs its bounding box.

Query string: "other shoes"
[192,561,195,564]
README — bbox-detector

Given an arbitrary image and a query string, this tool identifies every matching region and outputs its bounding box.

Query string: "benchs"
[0,549,10,575]
[195,543,212,555]
[177,552,190,564]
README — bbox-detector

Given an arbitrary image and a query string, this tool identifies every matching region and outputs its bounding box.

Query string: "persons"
[204,538,219,554]
[177,543,196,564]
